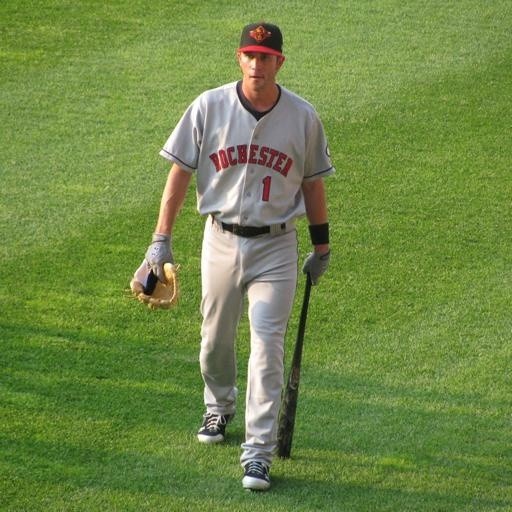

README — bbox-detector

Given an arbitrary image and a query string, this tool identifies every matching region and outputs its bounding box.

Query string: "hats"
[237,22,284,57]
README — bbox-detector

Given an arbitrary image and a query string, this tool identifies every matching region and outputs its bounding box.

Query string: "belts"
[222,222,285,238]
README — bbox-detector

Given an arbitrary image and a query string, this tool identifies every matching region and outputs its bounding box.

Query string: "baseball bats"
[275,272,312,459]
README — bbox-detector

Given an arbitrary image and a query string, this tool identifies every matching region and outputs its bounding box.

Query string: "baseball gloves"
[130,262,180,309]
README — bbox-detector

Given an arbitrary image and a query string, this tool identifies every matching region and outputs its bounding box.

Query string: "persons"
[145,23,337,491]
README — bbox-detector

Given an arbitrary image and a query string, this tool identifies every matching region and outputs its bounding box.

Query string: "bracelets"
[307,223,330,245]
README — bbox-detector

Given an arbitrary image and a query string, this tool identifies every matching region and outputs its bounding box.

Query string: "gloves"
[301,248,331,287]
[145,235,175,284]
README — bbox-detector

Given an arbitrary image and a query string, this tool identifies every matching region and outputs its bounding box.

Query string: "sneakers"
[242,460,271,491]
[197,413,235,442]
